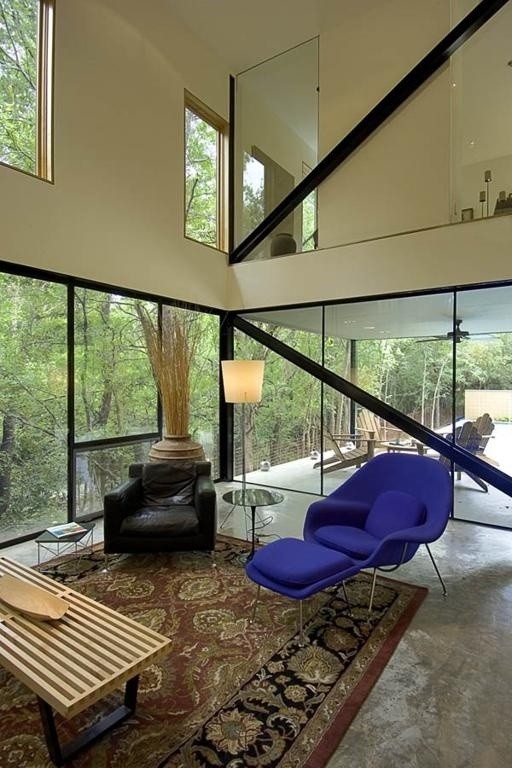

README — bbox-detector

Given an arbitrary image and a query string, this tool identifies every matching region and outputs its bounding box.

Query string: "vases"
[271,233,297,255]
[147,435,206,462]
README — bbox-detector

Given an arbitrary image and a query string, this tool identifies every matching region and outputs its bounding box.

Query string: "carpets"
[0,532,429,768]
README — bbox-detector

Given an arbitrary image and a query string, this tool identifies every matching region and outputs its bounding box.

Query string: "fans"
[414,319,500,344]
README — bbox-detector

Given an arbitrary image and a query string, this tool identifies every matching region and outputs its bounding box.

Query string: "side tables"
[34,522,98,577]
[221,488,285,546]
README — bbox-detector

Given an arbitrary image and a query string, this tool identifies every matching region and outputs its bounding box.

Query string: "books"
[46,522,88,539]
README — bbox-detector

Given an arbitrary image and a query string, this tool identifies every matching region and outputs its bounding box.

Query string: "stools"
[245,537,361,648]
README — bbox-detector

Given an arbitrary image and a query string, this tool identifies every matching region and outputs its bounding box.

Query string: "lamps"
[219,359,274,538]
[480,190,485,218]
[484,170,492,217]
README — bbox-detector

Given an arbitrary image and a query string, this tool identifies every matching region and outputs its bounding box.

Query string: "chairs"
[103,460,216,554]
[302,452,452,613]
[313,406,495,492]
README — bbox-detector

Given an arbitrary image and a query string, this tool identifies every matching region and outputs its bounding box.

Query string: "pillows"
[140,457,197,507]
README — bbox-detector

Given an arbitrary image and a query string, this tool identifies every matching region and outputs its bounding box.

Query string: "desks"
[0,555,173,767]
